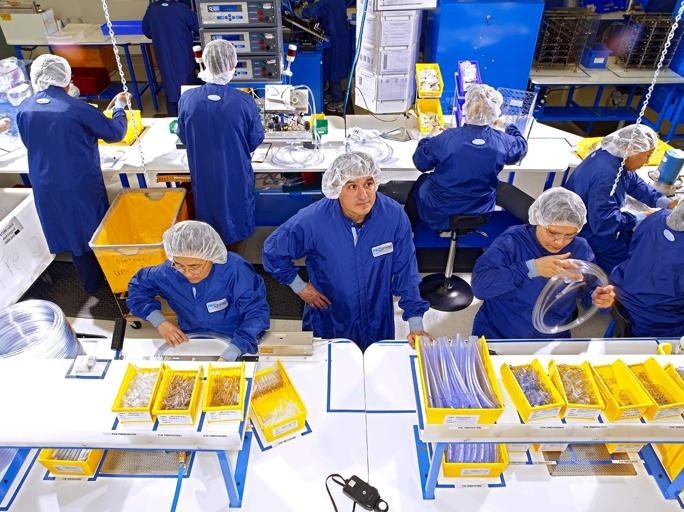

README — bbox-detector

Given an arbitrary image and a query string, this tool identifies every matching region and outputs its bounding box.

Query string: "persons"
[301,0,353,112]
[121,218,271,363]
[260,150,438,351]
[603,194,684,337]
[16,53,128,301]
[553,123,658,273]
[466,185,620,339]
[172,38,261,248]
[141,0,199,117]
[397,81,529,233]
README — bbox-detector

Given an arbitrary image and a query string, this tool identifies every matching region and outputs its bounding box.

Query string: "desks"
[1,24,684,512]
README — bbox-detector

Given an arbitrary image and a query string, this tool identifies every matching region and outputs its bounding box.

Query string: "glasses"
[171,260,207,275]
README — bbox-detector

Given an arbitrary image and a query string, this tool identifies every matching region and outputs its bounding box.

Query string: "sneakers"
[323,97,345,113]
